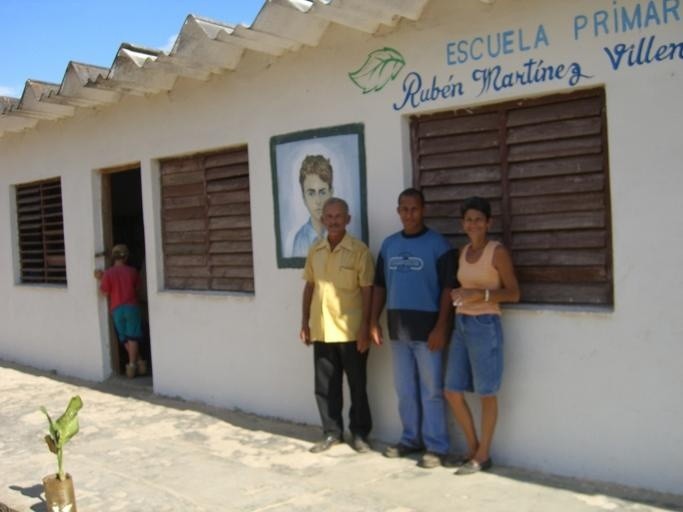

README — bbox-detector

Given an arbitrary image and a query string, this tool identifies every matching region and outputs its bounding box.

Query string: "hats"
[112,245,129,257]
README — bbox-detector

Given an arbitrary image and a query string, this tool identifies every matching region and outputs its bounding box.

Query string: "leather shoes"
[311,436,343,454]
[351,435,370,453]
[449,455,493,474]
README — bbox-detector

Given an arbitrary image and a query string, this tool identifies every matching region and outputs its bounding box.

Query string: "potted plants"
[39,396,83,511]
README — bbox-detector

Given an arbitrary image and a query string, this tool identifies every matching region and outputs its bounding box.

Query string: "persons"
[368,188,461,468]
[292,155,335,257]
[94,243,147,379]
[442,197,522,475]
[299,197,376,453]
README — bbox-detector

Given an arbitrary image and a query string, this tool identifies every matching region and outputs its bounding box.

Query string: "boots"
[126,361,136,378]
[137,359,147,374]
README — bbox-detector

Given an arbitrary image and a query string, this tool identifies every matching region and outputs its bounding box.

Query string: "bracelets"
[484,289,490,302]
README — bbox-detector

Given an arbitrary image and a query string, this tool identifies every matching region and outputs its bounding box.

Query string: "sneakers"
[421,453,445,467]
[384,444,417,458]
